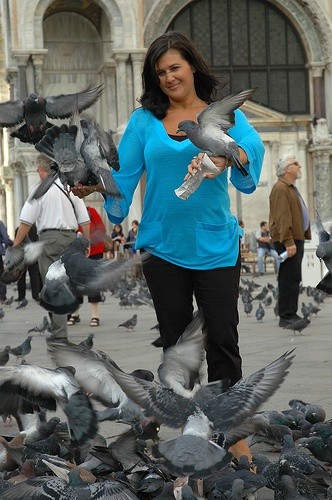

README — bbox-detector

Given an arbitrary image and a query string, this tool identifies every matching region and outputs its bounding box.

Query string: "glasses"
[284,161,298,170]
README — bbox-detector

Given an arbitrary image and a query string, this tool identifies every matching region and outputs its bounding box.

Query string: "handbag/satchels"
[104,234,113,253]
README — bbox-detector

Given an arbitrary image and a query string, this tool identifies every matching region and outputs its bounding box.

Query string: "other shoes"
[259,272,264,276]
[14,298,25,302]
[279,315,303,327]
[217,433,257,475]
[32,296,40,301]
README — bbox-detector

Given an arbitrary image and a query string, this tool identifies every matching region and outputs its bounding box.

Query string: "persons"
[269,153,312,326]
[256,221,281,273]
[68,206,106,327]
[0,220,14,303]
[69,32,266,475]
[237,220,247,252]
[14,154,91,345]
[106,220,141,258]
[15,222,43,301]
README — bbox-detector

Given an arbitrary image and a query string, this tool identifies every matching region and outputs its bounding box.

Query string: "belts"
[43,228,75,233]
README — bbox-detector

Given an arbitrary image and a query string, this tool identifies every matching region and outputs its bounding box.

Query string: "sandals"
[90,318,99,327]
[66,314,80,325]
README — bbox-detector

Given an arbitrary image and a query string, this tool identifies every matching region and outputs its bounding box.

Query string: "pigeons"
[0,81,106,144]
[0,116,332,500]
[176,86,257,176]
[97,346,298,480]
[36,236,154,315]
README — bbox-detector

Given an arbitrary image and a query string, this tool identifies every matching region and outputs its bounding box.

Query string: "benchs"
[241,252,277,272]
